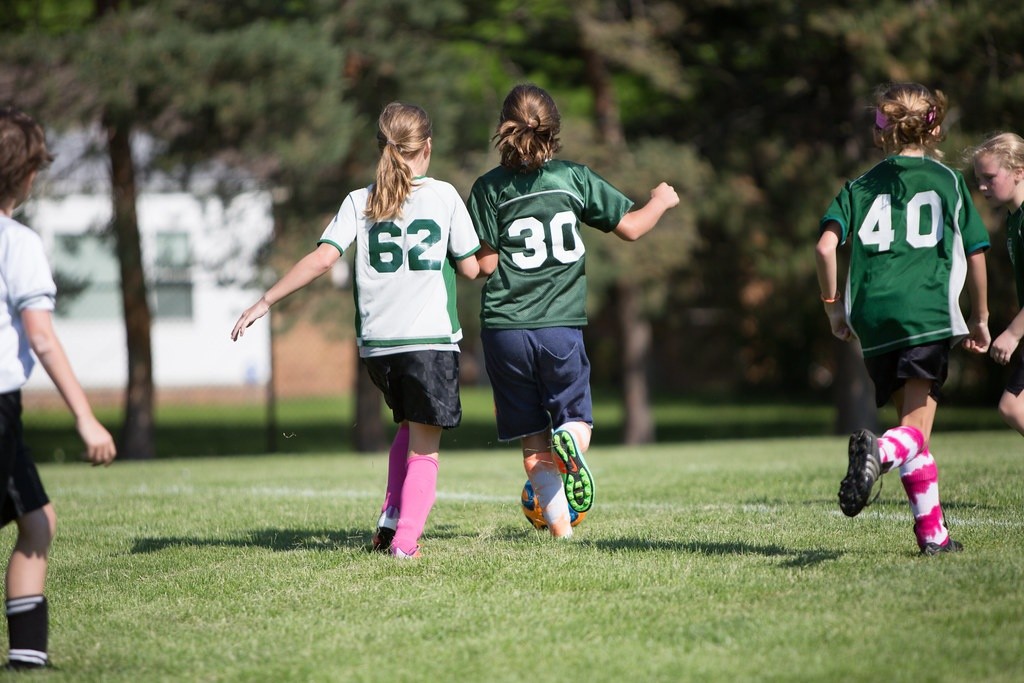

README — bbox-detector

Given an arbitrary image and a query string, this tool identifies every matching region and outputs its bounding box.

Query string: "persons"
[816,81,992,555]
[969,132,1024,437]
[0,94,120,674]
[229,101,482,564]
[464,84,680,539]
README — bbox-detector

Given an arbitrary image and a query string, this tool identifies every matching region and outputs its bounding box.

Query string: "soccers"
[521,473,589,532]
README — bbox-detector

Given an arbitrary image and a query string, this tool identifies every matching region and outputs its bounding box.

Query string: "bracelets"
[820,291,841,303]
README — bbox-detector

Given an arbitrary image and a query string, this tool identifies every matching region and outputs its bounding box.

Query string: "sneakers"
[551,429,595,513]
[838,429,894,517]
[373,506,400,554]
[390,544,423,561]
[918,538,964,557]
